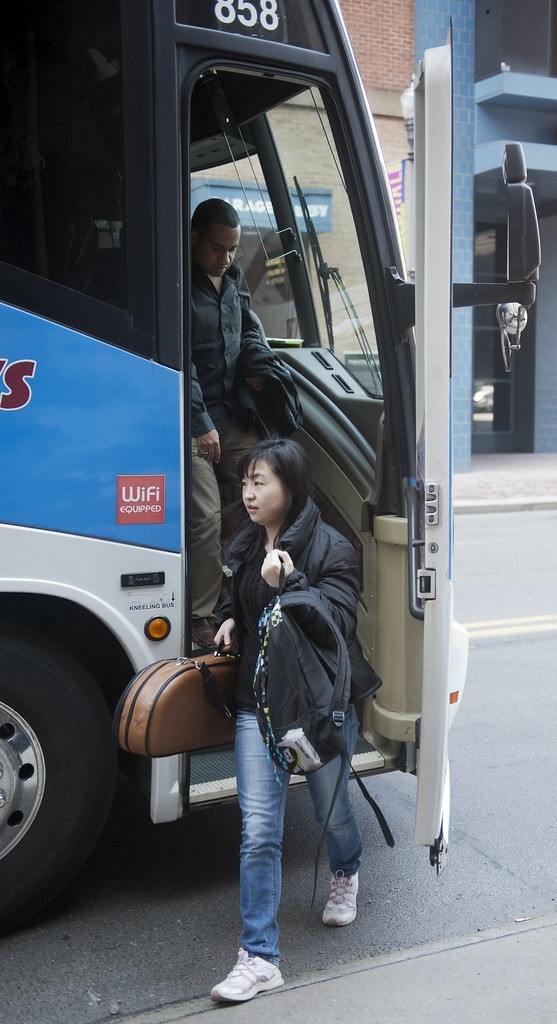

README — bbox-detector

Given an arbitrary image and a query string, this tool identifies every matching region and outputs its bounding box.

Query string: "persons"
[209,439,383,1001]
[186,199,302,652]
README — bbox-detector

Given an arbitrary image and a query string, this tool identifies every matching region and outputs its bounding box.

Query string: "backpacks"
[251,557,351,774]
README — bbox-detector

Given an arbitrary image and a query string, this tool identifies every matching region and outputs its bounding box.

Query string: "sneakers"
[322,869,359,926]
[211,947,285,1001]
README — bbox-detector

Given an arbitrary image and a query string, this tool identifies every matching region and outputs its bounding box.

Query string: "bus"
[0,2,544,935]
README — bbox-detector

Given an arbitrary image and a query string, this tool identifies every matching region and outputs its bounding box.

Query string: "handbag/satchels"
[110,648,238,757]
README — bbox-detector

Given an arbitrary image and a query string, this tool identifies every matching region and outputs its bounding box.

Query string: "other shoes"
[189,618,218,649]
